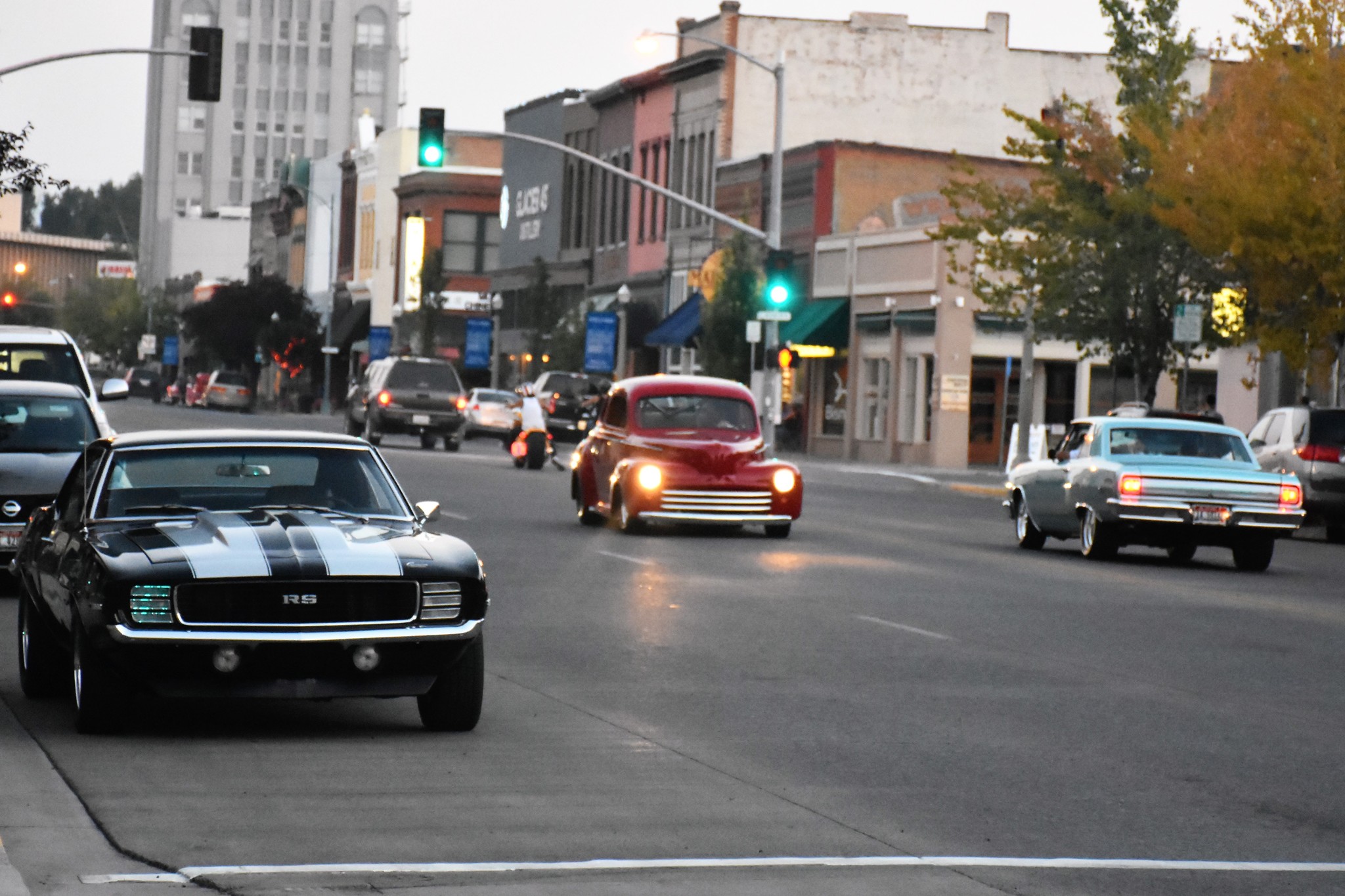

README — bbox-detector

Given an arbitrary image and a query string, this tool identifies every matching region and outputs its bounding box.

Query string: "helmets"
[522,383,535,397]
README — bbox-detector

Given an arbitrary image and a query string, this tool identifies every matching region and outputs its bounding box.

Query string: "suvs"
[1231,400,1345,548]
[1103,400,1236,459]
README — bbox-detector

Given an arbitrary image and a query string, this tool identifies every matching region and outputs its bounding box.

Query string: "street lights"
[637,30,786,458]
[611,282,633,382]
[488,292,504,390]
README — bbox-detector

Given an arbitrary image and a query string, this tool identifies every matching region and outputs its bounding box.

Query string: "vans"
[0,320,129,414]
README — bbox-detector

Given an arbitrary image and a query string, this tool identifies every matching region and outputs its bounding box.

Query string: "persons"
[508,385,566,471]
[1197,394,1224,426]
[1297,396,1311,409]
[576,378,616,441]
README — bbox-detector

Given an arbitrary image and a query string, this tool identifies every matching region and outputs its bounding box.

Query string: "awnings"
[643,293,707,347]
[778,296,851,347]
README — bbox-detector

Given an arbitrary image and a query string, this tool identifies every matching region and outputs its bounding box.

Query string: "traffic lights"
[777,347,799,368]
[763,249,792,310]
[417,107,446,169]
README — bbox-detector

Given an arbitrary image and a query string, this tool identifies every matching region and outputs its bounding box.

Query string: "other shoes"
[552,457,565,471]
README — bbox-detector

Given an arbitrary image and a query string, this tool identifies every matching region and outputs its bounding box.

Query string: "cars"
[122,366,255,414]
[0,377,133,597]
[340,353,614,472]
[1003,416,1309,574]
[569,371,805,540]
[7,426,489,733]
